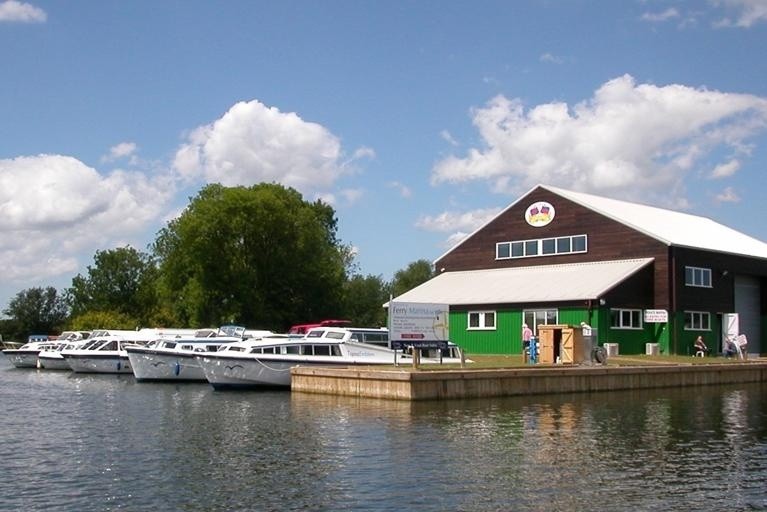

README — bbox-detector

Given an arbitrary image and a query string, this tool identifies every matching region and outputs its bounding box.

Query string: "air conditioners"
[645,342,661,357]
[602,342,619,357]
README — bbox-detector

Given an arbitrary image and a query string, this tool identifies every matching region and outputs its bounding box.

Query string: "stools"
[695,350,704,358]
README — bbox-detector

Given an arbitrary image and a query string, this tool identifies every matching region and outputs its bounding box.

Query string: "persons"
[733,337,741,354]
[693,335,710,358]
[522,324,532,349]
[723,339,736,358]
